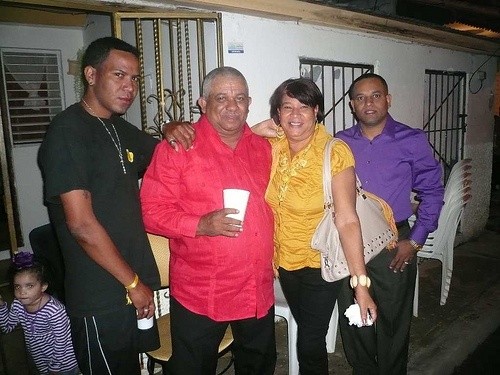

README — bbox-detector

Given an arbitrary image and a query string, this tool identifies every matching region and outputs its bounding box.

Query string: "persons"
[139,66,271,375]
[0,251,83,375]
[37,36,197,375]
[250,78,378,375]
[335,73,445,375]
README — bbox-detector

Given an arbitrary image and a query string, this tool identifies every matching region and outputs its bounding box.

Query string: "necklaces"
[82,98,127,174]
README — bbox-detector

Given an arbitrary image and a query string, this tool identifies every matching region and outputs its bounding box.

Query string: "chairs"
[29,223,65,300]
[145,233,233,375]
[274,277,339,375]
[408,158,473,318]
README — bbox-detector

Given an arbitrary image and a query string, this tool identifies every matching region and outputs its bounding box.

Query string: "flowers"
[13,251,33,270]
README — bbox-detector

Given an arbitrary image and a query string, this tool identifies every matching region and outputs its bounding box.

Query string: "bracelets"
[124,273,139,306]
[349,275,371,289]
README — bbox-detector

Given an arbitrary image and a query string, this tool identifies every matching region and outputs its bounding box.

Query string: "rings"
[404,261,408,264]
[143,308,148,311]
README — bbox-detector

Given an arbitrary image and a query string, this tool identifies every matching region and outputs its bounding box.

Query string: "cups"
[136,309,154,329]
[222,188,250,228]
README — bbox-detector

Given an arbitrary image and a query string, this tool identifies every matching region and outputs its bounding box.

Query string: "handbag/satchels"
[309,138,396,283]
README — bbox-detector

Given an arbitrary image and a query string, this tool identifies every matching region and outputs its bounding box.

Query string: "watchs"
[408,239,424,252]
